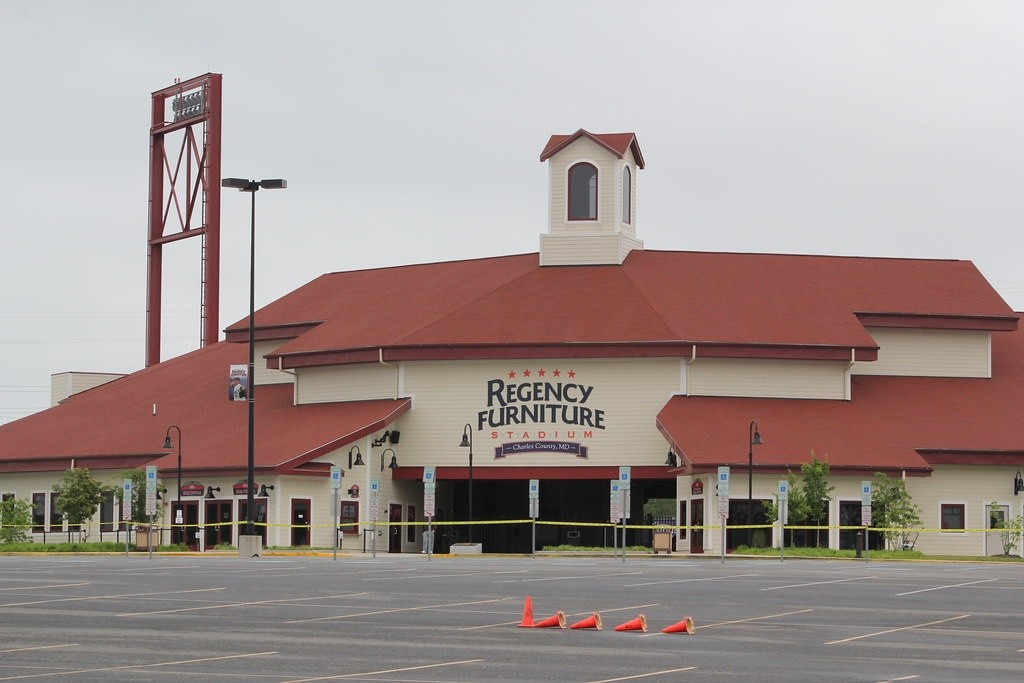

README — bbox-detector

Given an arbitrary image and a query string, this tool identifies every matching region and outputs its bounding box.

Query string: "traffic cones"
[569,613,604,631]
[662,615,696,636]
[517,596,535,627]
[614,614,648,633]
[533,611,566,628]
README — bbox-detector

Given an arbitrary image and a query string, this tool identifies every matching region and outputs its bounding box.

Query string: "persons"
[233,377,245,401]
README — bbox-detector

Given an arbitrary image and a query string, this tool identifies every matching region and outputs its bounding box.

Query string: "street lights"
[458,424,474,545]
[746,420,764,548]
[218,176,291,561]
[162,425,182,545]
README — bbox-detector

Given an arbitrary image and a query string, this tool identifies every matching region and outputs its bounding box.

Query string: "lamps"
[259,484,275,497]
[155,488,167,499]
[1014,471,1024,495]
[380,448,398,472]
[348,445,365,469]
[665,446,677,469]
[203,487,221,498]
